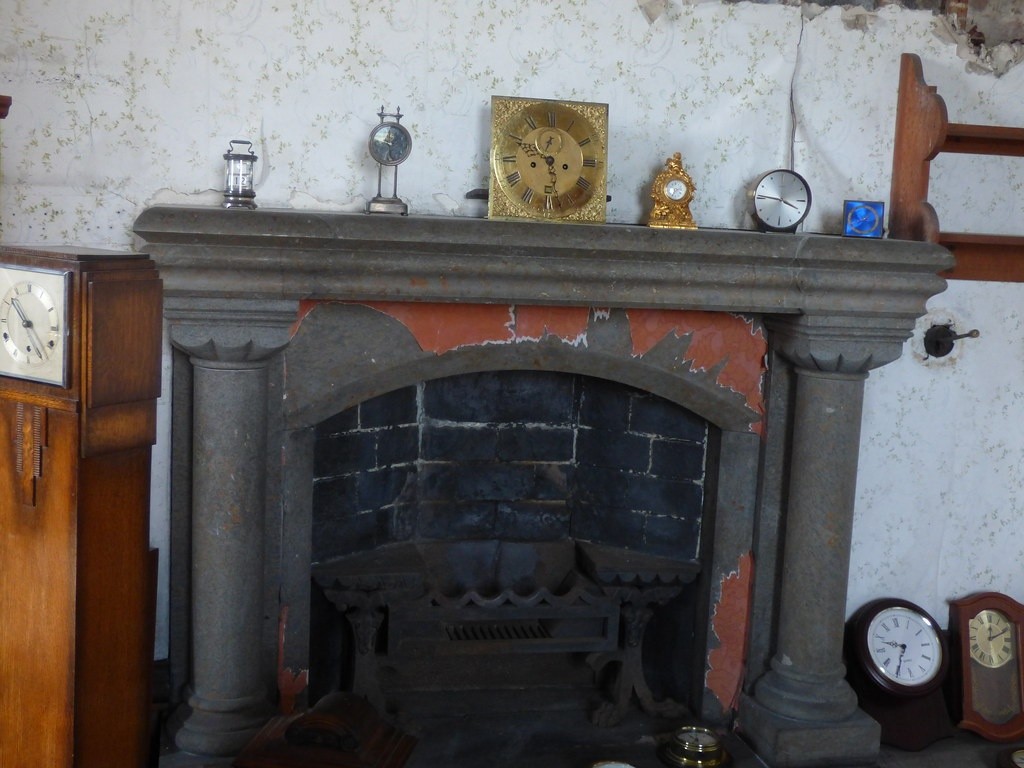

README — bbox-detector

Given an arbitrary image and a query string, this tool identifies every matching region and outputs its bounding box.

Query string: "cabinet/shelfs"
[888,53,1024,282]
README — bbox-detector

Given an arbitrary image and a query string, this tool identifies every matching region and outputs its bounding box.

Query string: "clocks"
[996,745,1024,768]
[842,200,886,238]
[646,151,698,232]
[661,725,726,768]
[843,598,951,753]
[948,593,1024,743]
[487,96,610,225]
[0,263,72,390]
[748,168,812,234]
[364,105,412,214]
